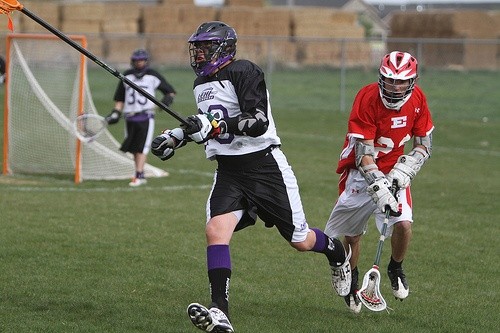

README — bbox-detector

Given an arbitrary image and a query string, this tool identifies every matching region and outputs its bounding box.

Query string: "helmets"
[188,21,237,77]
[378,51,418,110]
[131,49,148,77]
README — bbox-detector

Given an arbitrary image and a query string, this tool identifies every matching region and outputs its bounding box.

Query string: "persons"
[324,50,435,314]
[151,21,352,333]
[105,48,177,187]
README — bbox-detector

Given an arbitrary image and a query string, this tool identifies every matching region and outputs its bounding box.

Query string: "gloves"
[151,127,187,161]
[180,112,227,145]
[159,95,173,111]
[0,75,4,84]
[387,154,421,190]
[106,109,121,125]
[366,177,402,217]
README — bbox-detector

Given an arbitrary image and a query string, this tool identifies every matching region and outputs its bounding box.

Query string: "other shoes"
[129,176,147,186]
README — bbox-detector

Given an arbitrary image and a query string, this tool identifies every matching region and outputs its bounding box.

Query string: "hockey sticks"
[72,108,157,143]
[355,182,398,316]
[0,0,192,128]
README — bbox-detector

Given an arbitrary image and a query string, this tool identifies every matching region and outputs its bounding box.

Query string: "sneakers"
[387,262,410,301]
[187,303,235,333]
[344,268,362,313]
[329,237,352,296]
